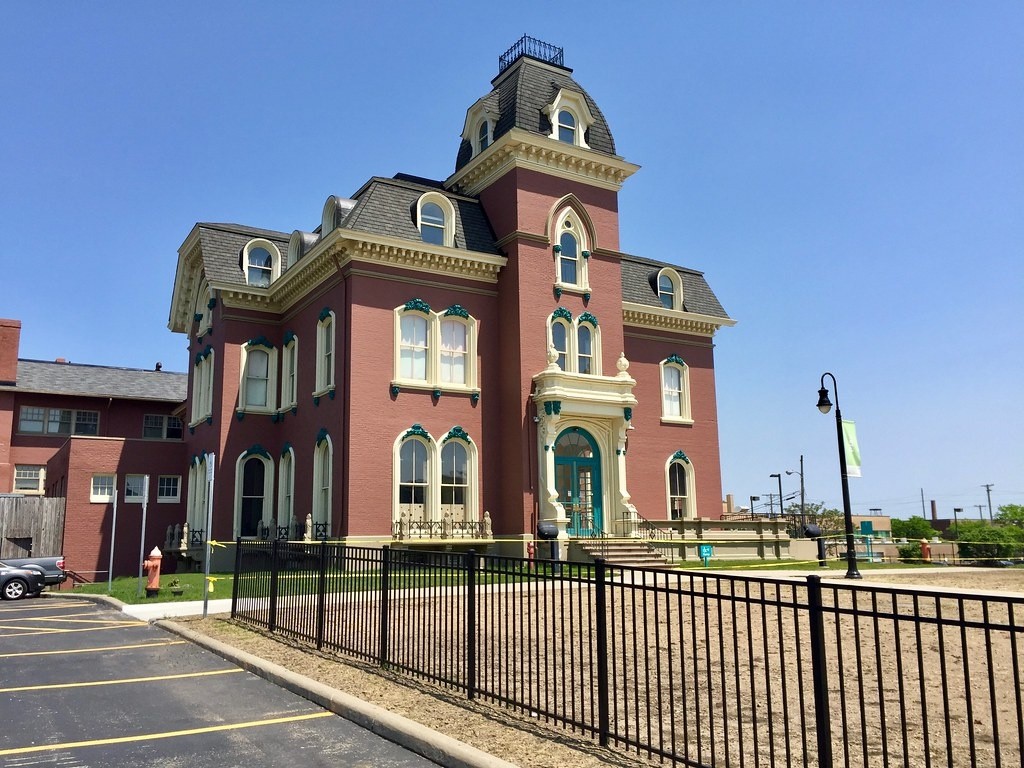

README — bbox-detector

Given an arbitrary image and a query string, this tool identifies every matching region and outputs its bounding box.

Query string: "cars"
[996,560,1015,569]
[0,561,45,599]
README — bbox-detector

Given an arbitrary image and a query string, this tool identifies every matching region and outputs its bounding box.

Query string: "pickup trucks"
[0,556,67,599]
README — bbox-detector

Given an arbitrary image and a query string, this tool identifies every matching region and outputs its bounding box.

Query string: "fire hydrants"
[142,546,163,599]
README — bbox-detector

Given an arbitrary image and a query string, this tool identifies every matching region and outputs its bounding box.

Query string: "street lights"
[815,371,864,579]
[954,507,964,541]
[786,470,805,538]
[769,474,783,518]
[750,496,759,521]
